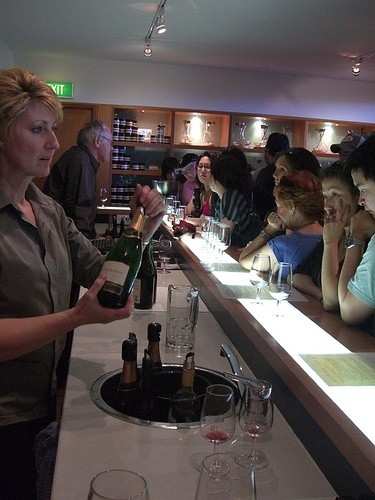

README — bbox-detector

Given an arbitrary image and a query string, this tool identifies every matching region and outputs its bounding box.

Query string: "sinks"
[91,363,244,430]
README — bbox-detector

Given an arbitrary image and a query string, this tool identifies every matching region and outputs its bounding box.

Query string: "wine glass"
[156,240,173,274]
[88,469,150,500]
[200,216,232,271]
[194,452,257,500]
[199,384,237,478]
[99,187,108,207]
[166,197,174,210]
[178,205,188,221]
[248,253,272,307]
[172,200,181,214]
[268,262,293,317]
[232,378,274,472]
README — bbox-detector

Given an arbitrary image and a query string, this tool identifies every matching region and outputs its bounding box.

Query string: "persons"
[252,132,290,227]
[40,118,115,242]
[0,66,169,500]
[329,131,368,163]
[286,159,371,312]
[207,154,265,247]
[161,150,220,231]
[218,145,271,227]
[238,168,325,288]
[337,131,375,326]
[272,146,321,187]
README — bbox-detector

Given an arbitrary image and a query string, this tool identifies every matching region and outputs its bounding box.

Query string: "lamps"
[143,38,153,58]
[352,58,363,76]
[156,6,166,35]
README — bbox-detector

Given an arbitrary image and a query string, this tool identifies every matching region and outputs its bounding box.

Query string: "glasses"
[100,134,114,145]
[284,147,305,170]
[279,175,294,188]
[197,165,211,169]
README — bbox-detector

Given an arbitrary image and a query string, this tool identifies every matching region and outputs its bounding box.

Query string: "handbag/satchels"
[232,213,265,248]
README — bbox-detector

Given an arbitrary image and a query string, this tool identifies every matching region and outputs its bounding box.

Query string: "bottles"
[131,242,156,310]
[114,331,138,418]
[112,216,120,246]
[167,351,200,422]
[150,245,157,304]
[119,218,125,238]
[134,348,164,424]
[97,202,144,309]
[140,322,165,421]
[106,216,115,252]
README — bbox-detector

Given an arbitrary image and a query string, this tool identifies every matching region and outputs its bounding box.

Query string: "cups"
[164,284,200,353]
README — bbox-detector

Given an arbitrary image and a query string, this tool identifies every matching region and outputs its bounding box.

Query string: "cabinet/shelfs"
[109,105,375,215]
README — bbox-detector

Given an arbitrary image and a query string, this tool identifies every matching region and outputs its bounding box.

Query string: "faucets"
[219,343,266,416]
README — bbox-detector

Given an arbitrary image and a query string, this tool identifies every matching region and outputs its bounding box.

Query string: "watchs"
[344,235,367,250]
[259,230,273,244]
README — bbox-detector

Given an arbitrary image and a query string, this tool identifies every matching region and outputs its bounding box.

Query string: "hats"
[330,133,366,153]
[175,153,198,174]
